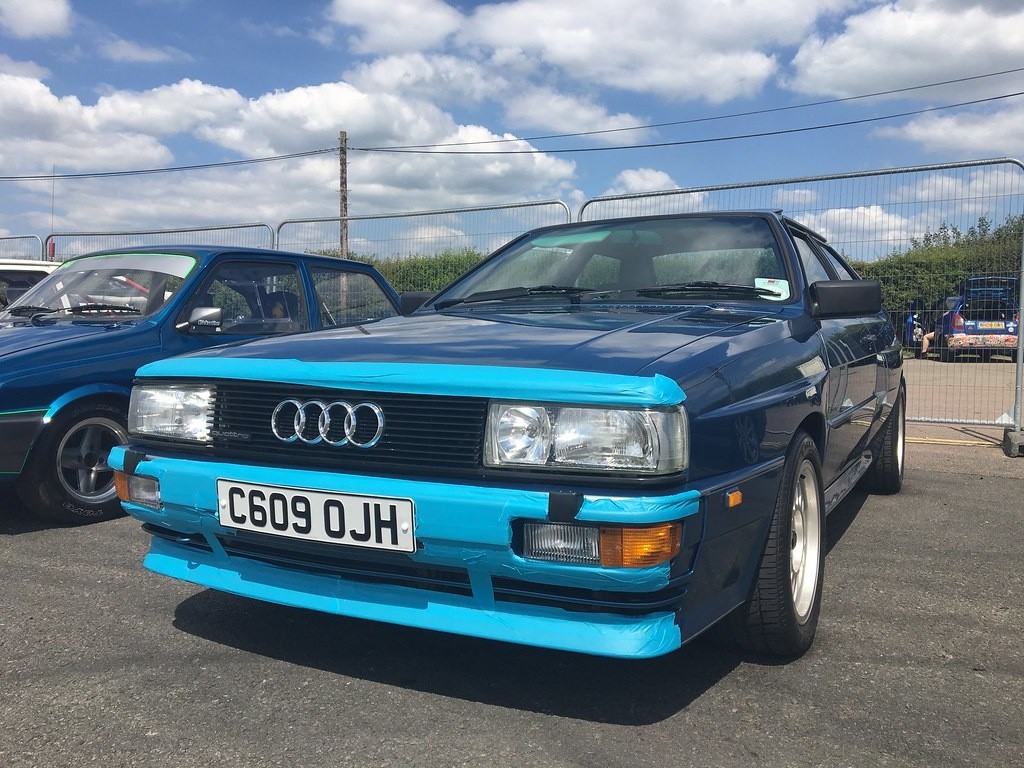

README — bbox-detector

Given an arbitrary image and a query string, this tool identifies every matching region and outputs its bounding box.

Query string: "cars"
[0,259,173,319]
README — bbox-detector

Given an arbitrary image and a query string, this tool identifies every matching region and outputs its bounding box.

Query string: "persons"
[916,332,935,358]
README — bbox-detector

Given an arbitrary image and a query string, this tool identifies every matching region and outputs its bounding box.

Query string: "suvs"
[0,244,403,527]
[107,205,909,667]
[905,276,1024,362]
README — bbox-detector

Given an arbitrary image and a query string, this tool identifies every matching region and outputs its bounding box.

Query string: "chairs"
[227,291,299,332]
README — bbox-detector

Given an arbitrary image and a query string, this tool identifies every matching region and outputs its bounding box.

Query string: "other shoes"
[917,353,928,359]
